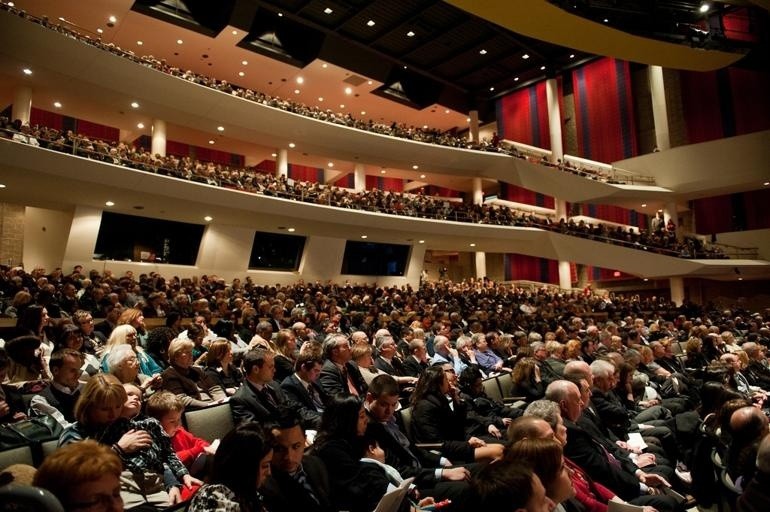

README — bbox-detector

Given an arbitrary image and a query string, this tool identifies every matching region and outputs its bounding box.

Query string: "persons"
[292,102,469,150]
[346,186,515,227]
[181,70,299,114]
[471,130,622,185]
[1,261,769,510]
[511,209,732,261]
[3,1,68,35]
[65,27,182,78]
[189,160,352,211]
[1,116,200,183]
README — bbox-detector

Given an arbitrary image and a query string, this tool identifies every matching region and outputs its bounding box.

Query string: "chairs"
[0,276,768,511]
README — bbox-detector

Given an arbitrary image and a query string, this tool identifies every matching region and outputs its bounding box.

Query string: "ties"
[342,366,360,396]
[307,384,326,409]
[262,388,278,406]
[593,438,623,469]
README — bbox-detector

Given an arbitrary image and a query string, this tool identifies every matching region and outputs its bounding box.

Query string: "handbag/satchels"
[0,409,65,451]
